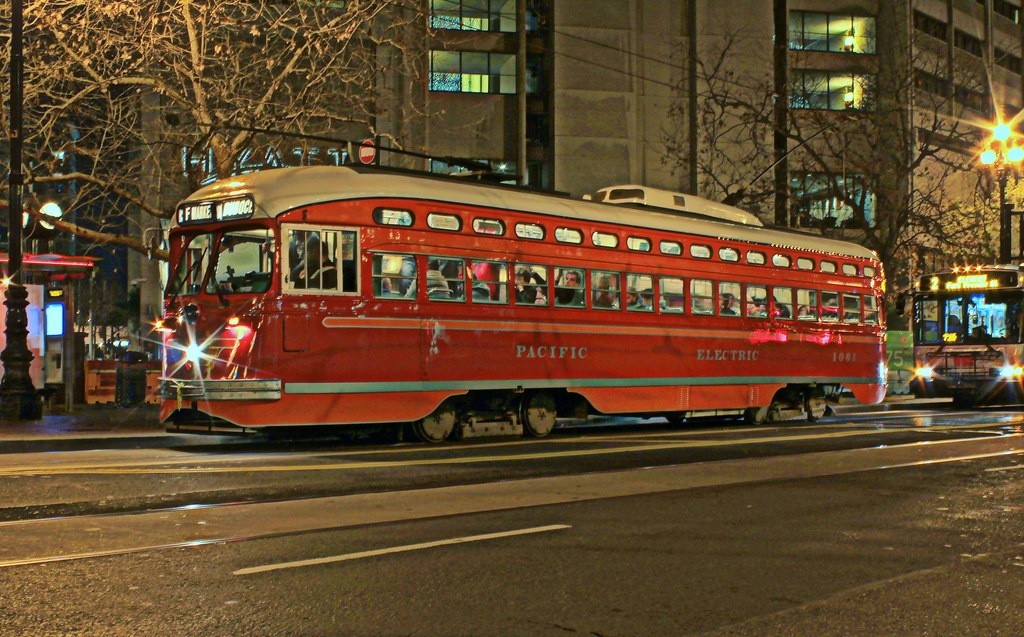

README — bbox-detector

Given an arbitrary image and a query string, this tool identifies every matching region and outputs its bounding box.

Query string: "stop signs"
[359,140,375,163]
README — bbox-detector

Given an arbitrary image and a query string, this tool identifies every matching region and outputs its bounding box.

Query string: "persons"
[946,313,988,343]
[259,232,877,320]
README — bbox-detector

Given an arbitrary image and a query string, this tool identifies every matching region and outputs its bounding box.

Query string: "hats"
[829,299,837,305]
[557,289,575,303]
[641,288,652,298]
[303,235,328,251]
[627,287,636,294]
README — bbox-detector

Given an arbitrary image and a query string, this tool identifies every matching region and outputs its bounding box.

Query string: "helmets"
[512,269,530,282]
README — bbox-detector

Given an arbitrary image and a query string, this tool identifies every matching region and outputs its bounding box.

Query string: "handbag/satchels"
[535,288,546,304]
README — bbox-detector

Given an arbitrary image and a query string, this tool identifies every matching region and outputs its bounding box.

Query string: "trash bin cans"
[114,350,149,405]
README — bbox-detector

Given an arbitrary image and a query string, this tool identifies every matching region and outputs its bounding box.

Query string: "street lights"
[981,124,1024,264]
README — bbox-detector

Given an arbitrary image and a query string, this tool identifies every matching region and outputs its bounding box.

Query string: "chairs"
[335,259,875,324]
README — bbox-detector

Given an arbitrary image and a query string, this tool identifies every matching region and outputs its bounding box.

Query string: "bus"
[142,165,889,445]
[895,264,1024,410]
